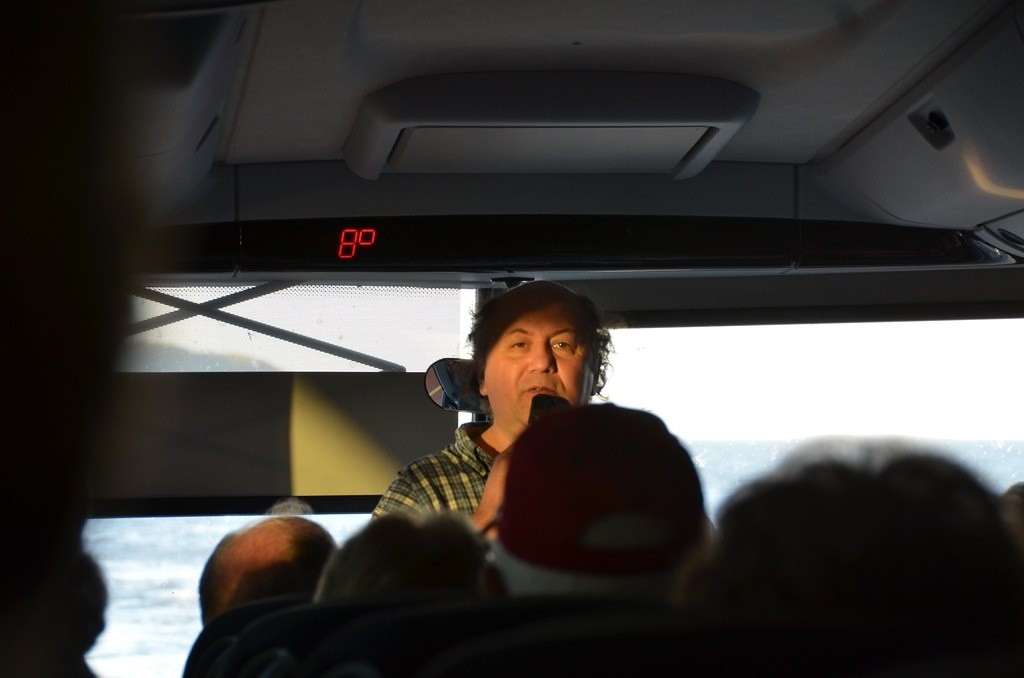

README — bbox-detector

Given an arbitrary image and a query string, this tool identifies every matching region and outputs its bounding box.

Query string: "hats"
[494,401,713,600]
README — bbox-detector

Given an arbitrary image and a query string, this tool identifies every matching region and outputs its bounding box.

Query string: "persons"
[367,280,719,574]
[314,508,506,609]
[198,514,338,627]
[488,402,708,605]
[694,443,1024,627]
[441,367,484,411]
